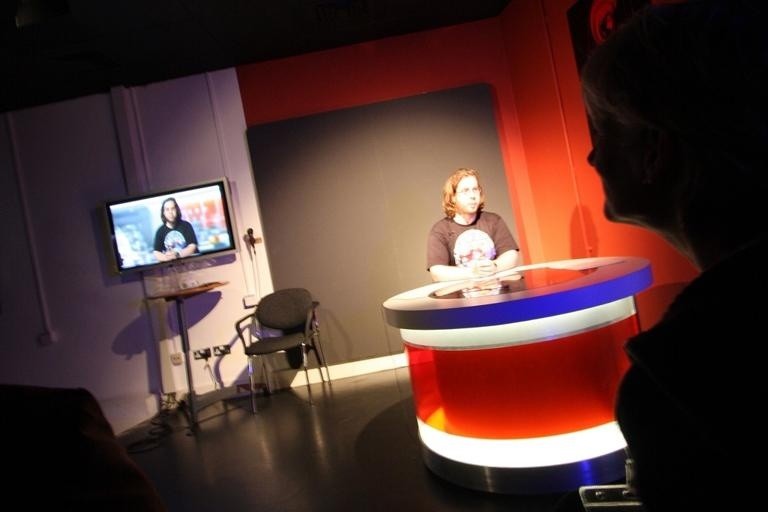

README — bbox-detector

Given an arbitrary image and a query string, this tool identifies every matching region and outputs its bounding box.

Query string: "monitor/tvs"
[102,176,241,277]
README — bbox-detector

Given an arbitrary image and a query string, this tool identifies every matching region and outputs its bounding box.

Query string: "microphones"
[247,227,255,249]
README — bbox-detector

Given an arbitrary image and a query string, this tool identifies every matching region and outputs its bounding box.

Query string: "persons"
[426,168,519,281]
[153,198,198,262]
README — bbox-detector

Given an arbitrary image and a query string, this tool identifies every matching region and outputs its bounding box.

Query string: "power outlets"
[213,345,231,356]
[194,348,211,360]
[171,352,182,364]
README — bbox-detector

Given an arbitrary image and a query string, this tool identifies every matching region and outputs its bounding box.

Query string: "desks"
[145,278,227,439]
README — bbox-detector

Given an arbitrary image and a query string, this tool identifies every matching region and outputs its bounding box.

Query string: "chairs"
[235,286,333,415]
